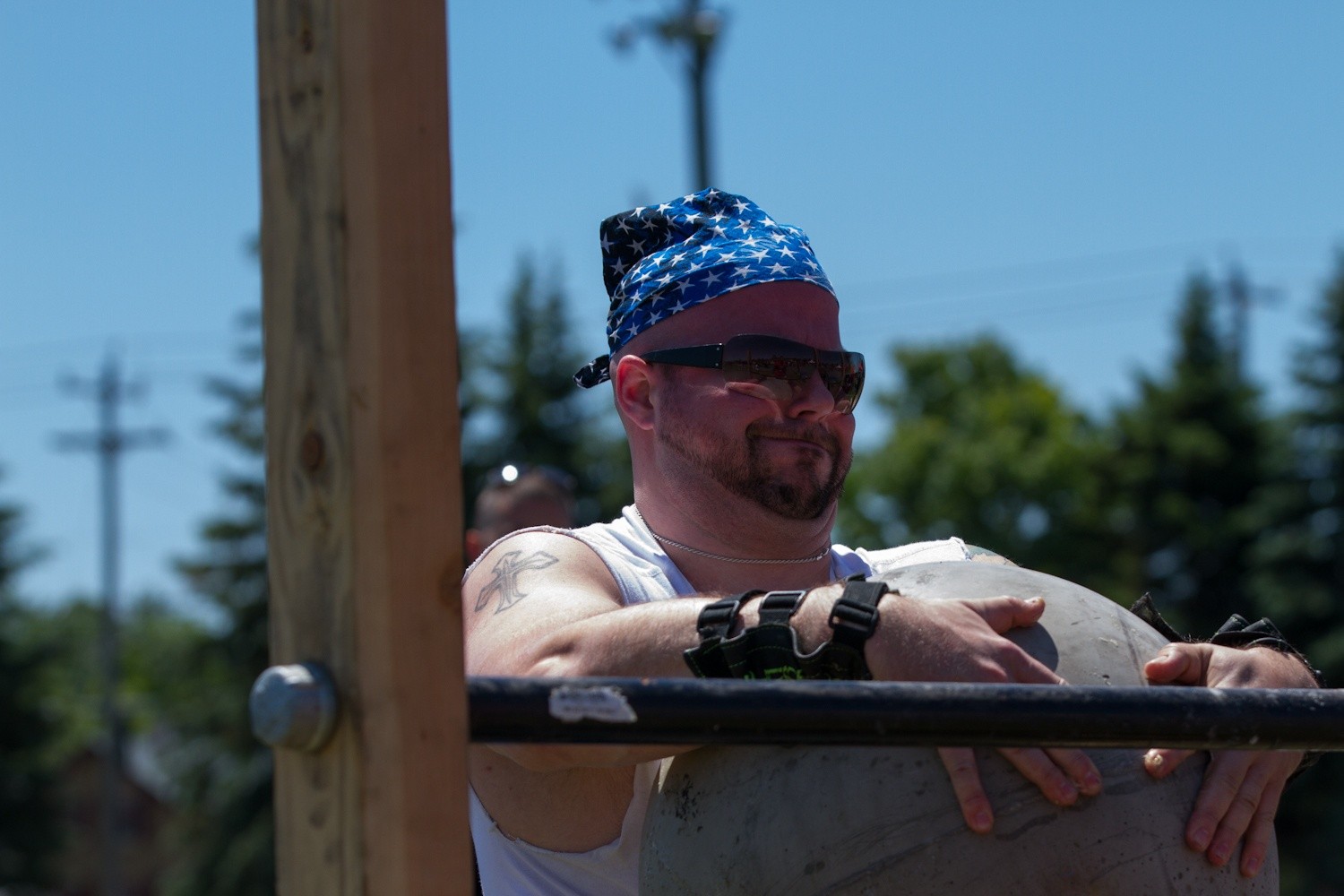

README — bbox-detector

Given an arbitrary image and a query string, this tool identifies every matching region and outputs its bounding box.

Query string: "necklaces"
[635,505,832,566]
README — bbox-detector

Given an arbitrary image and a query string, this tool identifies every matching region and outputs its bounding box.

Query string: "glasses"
[640,331,866,416]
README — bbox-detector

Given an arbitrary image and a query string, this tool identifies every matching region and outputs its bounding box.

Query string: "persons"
[461,184,1344,896]
[461,475,583,602]
